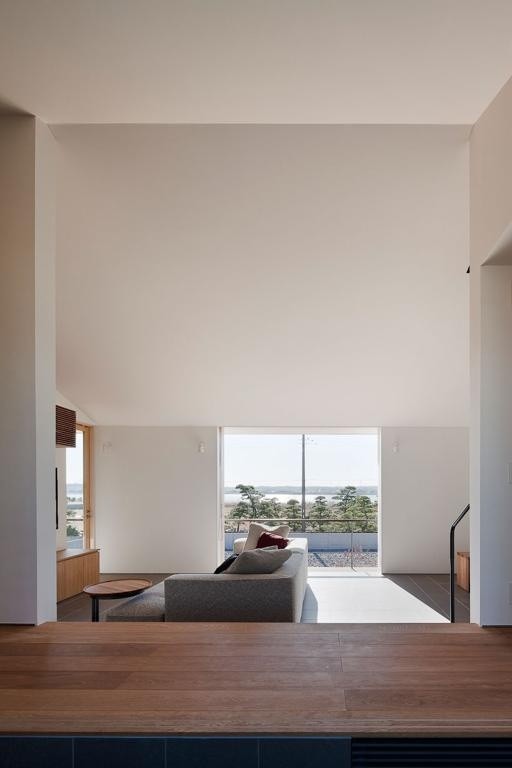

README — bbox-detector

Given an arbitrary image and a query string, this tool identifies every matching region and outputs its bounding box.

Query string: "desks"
[82,577,153,622]
[0,621,511,766]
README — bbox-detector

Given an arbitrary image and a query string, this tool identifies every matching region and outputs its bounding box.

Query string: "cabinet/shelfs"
[56,403,77,449]
[57,548,100,604]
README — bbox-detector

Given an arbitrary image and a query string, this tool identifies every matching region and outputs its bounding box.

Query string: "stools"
[455,551,470,593]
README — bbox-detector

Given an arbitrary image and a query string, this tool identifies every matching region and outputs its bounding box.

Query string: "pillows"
[223,522,294,575]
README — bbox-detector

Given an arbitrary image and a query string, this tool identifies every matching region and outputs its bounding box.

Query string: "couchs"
[103,537,309,624]
